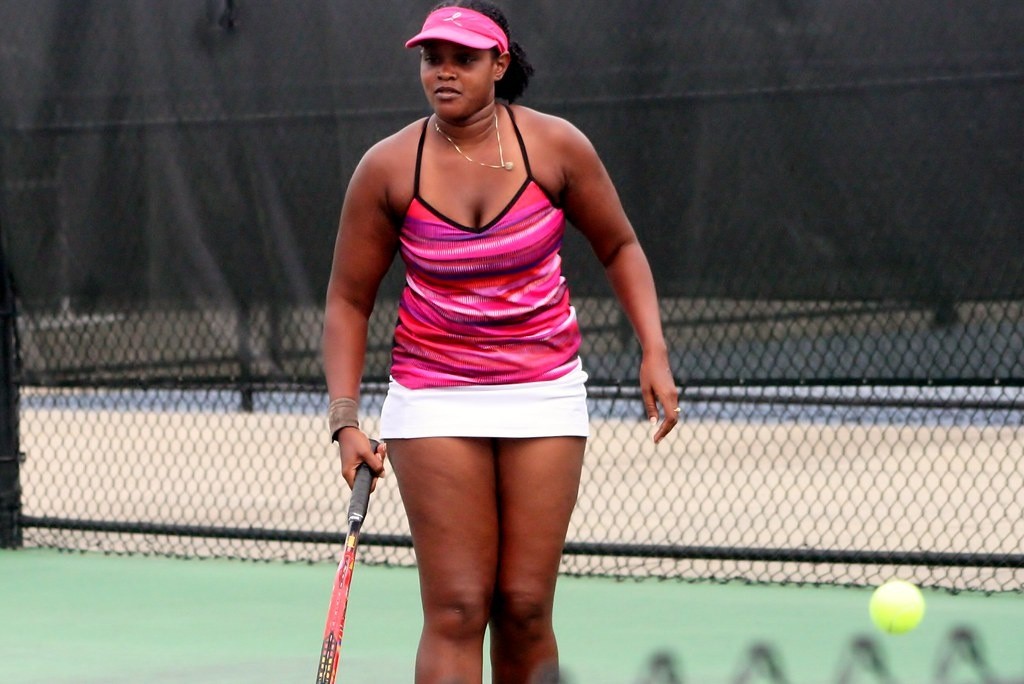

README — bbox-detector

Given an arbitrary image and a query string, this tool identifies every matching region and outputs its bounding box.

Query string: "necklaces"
[434,108,513,170]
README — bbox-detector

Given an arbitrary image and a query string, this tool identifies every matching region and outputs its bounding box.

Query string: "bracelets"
[328,398,359,443]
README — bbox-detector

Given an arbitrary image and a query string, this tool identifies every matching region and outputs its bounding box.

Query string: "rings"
[674,408,681,412]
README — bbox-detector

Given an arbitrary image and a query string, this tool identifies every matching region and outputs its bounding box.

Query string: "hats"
[405,7,509,54]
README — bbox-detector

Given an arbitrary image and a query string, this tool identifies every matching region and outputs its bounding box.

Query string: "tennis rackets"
[315,437,382,684]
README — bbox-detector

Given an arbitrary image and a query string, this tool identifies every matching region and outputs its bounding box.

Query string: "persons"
[320,1,682,684]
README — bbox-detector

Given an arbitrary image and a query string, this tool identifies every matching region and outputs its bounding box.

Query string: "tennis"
[868,578,926,634]
